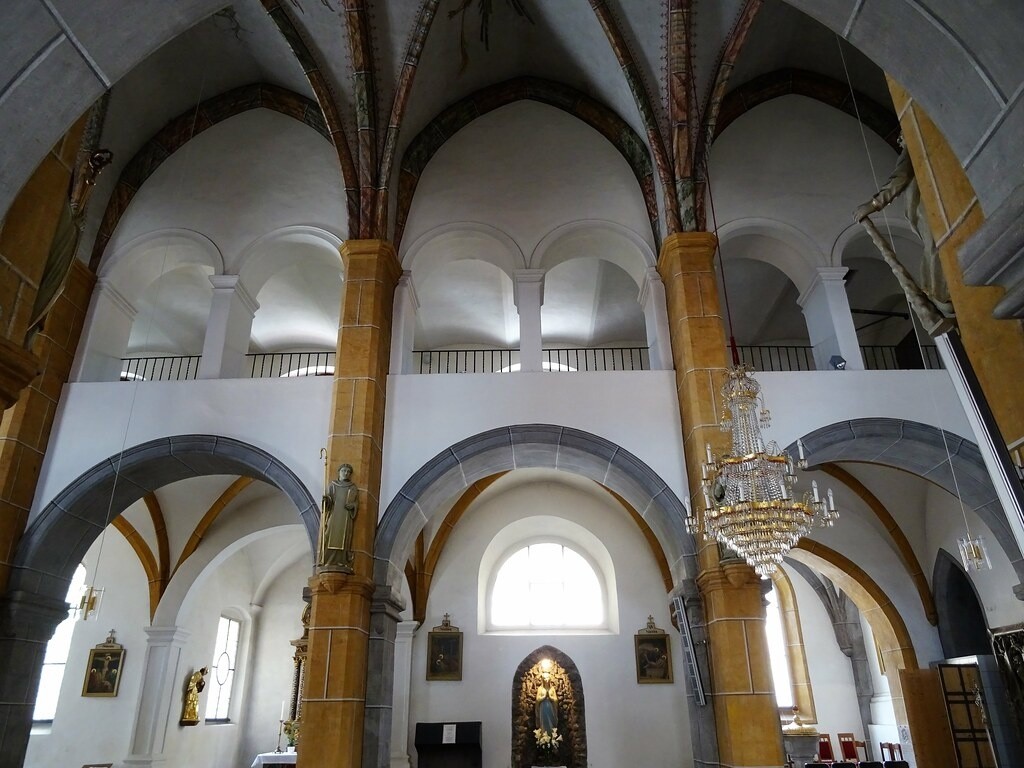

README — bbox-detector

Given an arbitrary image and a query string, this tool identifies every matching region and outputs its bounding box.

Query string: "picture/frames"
[424,631,463,680]
[634,634,674,684]
[80,649,126,697]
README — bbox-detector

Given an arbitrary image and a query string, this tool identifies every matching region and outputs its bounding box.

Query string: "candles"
[280,700,285,720]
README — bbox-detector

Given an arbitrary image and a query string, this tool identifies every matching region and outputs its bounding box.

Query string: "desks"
[415,720,483,768]
[250,751,297,768]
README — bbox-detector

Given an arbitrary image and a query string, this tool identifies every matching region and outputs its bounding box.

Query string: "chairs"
[807,733,909,768]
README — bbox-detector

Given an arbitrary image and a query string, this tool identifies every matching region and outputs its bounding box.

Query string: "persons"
[320,463,359,569]
[851,128,959,315]
[534,672,558,742]
[182,666,210,720]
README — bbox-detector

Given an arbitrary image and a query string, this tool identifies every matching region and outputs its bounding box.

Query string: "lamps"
[68,16,218,621]
[674,0,844,587]
[836,34,997,575]
